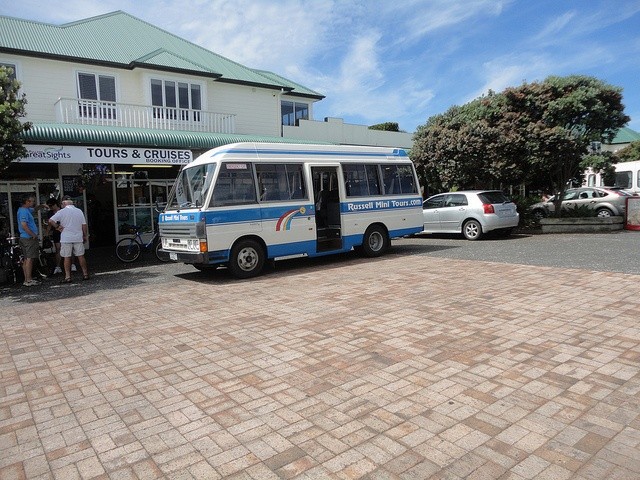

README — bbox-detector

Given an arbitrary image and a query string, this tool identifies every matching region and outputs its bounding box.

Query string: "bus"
[155,142,424,279]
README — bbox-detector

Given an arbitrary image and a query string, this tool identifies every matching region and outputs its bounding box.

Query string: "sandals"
[83,275,89,280]
[58,278,72,283]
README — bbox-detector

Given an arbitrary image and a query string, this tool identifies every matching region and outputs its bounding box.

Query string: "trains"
[575,161,639,196]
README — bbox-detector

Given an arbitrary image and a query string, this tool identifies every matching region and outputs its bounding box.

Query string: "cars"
[404,190,519,241]
[526,186,632,228]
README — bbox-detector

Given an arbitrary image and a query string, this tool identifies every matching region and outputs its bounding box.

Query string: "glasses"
[26,199,31,202]
[49,204,55,207]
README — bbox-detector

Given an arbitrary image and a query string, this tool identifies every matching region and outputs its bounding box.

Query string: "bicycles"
[4,233,49,287]
[115,201,170,263]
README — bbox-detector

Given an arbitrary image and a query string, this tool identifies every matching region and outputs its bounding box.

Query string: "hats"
[62,195,72,200]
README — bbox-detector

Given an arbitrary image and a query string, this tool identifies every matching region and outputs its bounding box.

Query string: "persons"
[45,198,78,274]
[16,195,50,287]
[47,196,90,283]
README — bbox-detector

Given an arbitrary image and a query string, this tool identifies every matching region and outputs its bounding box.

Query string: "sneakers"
[23,280,38,285]
[27,280,41,286]
[71,263,77,271]
[54,267,62,274]
